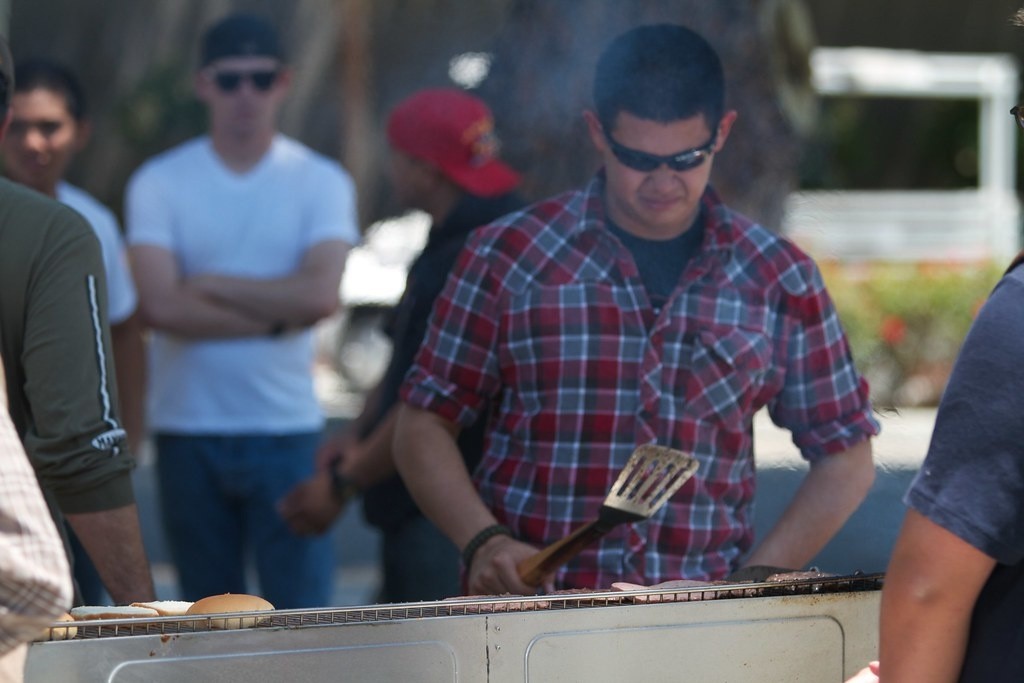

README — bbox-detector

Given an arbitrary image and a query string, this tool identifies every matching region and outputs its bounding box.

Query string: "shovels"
[517,443,700,583]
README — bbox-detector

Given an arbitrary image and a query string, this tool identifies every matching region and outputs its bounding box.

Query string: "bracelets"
[462,524,512,567]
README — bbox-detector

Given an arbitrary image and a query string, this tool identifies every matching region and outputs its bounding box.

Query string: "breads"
[30,594,274,641]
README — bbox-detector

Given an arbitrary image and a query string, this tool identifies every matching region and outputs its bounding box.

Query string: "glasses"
[603,129,716,172]
[215,68,280,92]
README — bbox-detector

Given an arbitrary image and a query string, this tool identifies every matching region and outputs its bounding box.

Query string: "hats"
[388,89,520,198]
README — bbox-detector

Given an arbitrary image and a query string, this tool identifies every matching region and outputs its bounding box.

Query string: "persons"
[391,23,882,599]
[124,14,361,608]
[842,253,1024,683]
[0,34,156,683]
[284,88,524,607]
